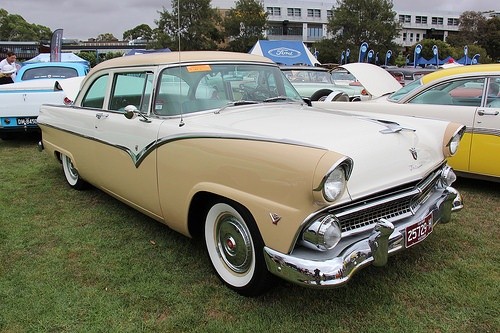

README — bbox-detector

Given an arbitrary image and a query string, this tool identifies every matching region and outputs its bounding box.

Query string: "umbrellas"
[409,56,475,66]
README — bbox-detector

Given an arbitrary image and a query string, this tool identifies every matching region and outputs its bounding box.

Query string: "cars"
[311,64,500,183]
[0,61,91,140]
[36,51,467,298]
[242,63,371,103]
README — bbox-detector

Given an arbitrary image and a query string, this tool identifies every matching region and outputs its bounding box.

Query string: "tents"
[247,39,321,67]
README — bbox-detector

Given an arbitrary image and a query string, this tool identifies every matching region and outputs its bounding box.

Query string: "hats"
[1,65,16,73]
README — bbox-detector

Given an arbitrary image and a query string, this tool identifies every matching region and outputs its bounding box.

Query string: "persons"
[0,52,17,84]
